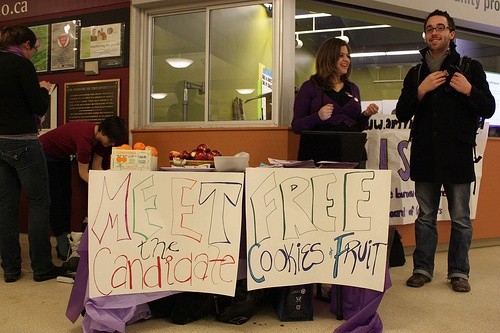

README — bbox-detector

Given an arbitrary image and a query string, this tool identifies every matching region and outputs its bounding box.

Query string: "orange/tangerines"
[117,142,159,156]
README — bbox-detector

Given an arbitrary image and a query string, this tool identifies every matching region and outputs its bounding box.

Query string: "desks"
[65,168,392,333]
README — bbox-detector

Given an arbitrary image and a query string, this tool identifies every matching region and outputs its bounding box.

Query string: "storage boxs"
[111,147,157,171]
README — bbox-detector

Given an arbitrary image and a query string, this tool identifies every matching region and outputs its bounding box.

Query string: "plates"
[160,167,215,172]
[320,162,359,169]
[170,159,214,166]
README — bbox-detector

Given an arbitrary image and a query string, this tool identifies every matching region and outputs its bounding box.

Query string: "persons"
[0,25,62,282]
[291,36,378,169]
[38,115,126,260]
[396,9,496,291]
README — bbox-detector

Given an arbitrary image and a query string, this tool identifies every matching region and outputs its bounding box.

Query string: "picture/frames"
[37,84,57,137]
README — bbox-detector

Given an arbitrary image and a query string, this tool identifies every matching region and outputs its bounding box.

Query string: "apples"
[169,143,221,161]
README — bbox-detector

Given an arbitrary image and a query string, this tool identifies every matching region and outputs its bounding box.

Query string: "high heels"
[56,246,69,261]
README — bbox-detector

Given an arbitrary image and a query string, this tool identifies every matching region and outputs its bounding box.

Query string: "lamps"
[165,50,193,69]
[335,31,349,44]
[295,34,303,48]
[235,88,255,95]
[151,93,168,99]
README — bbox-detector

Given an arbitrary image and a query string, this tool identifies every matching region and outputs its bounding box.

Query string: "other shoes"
[389,229,406,266]
[33,266,67,281]
[4,271,21,282]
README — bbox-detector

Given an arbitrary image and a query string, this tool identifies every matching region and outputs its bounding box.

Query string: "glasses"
[425,25,453,33]
[24,40,37,53]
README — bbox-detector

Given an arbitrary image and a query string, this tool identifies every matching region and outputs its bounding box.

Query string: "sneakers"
[407,273,431,287]
[451,277,470,292]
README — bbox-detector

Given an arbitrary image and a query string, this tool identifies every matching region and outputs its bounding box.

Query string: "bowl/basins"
[213,155,249,172]
